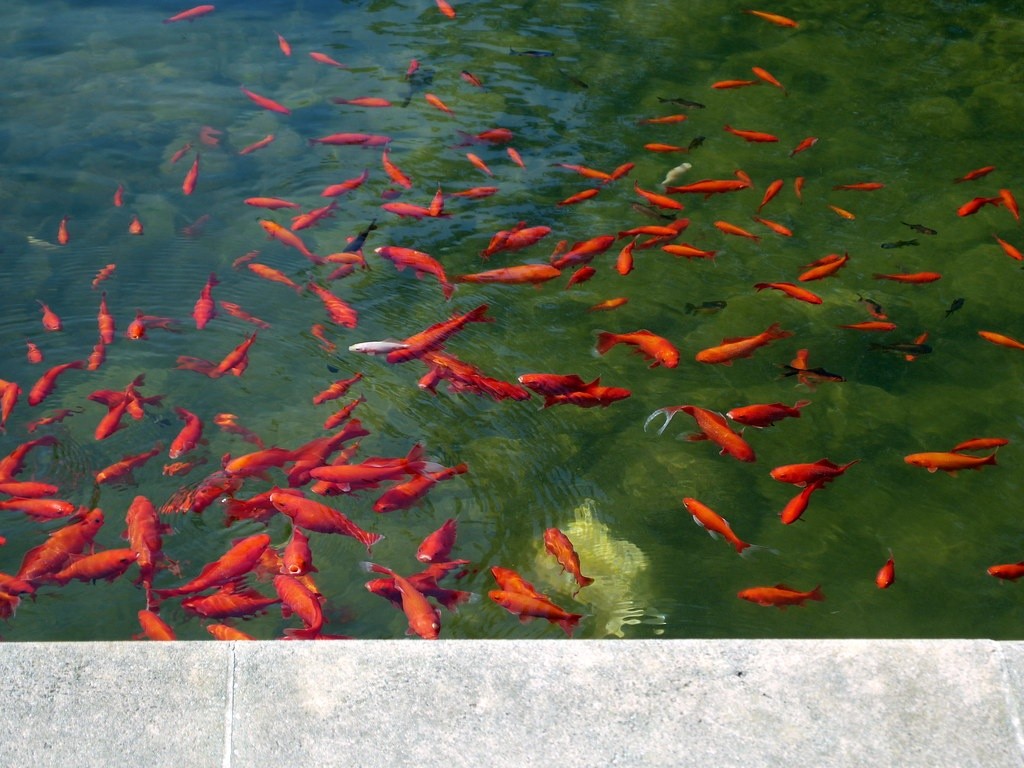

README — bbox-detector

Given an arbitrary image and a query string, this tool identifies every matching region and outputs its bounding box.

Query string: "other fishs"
[3,1,1024,638]
